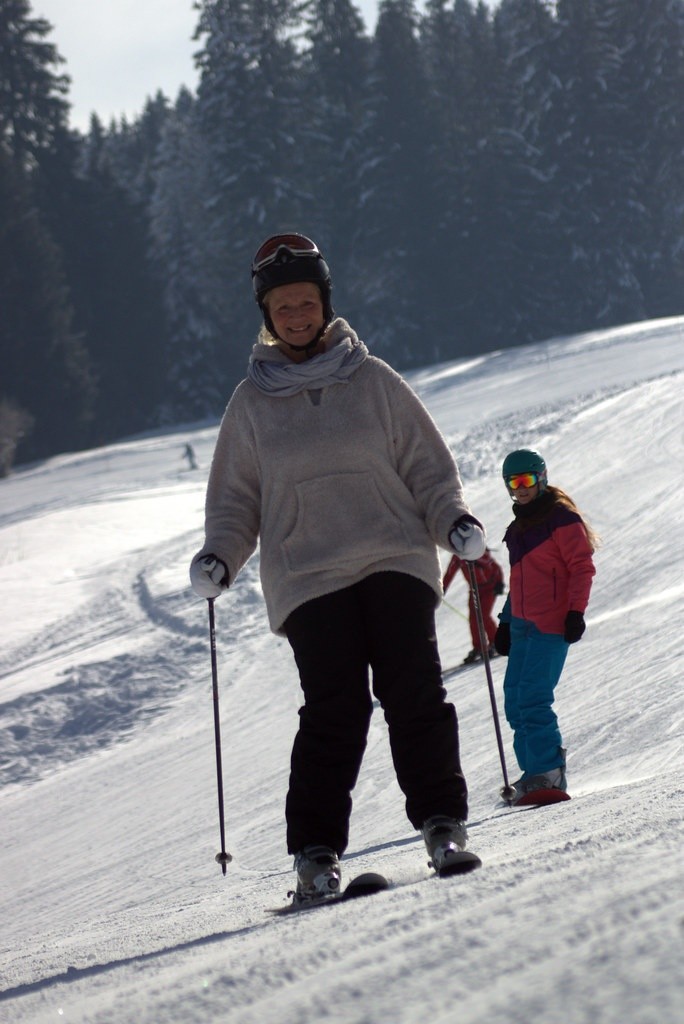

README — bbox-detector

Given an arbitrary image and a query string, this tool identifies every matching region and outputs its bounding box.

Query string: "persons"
[182,444,197,469]
[191,232,595,905]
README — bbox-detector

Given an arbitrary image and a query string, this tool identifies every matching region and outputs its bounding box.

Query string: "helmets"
[251,233,333,301]
[502,448,548,497]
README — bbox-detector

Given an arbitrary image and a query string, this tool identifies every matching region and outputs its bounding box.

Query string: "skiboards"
[263,871,389,912]
[438,850,482,880]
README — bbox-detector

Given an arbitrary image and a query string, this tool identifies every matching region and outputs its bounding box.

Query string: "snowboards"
[513,788,571,805]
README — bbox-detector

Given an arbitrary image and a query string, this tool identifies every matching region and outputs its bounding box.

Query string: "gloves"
[492,582,505,597]
[494,623,510,656]
[563,610,586,644]
[448,521,486,561]
[189,556,227,598]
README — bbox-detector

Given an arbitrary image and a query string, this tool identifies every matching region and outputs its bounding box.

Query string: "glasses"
[249,232,324,280]
[505,473,543,490]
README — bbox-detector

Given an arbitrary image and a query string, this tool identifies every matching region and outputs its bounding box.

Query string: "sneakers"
[287,844,340,905]
[487,643,501,657]
[423,815,483,879]
[464,651,483,664]
[499,748,567,804]
